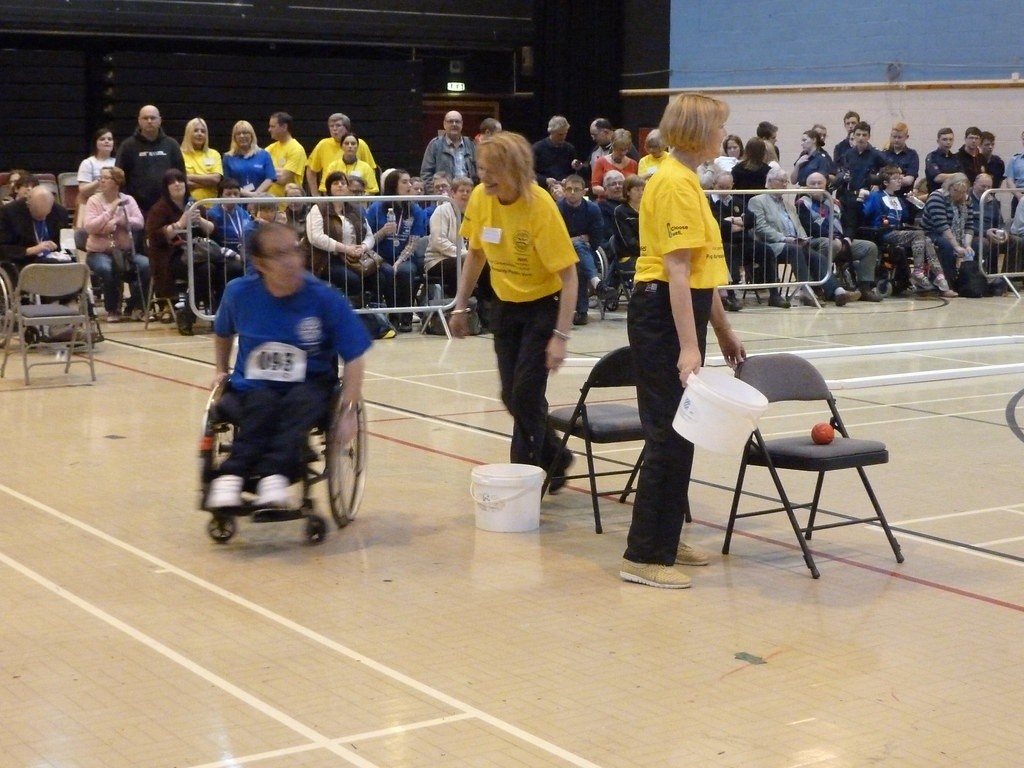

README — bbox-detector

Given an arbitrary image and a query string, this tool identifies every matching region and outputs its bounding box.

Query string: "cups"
[995,229,1004,237]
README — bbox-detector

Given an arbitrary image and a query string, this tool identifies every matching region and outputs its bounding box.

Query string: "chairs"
[540,345,694,535]
[855,210,918,290]
[603,234,634,312]
[0,263,97,386]
[720,352,907,578]
[143,238,210,331]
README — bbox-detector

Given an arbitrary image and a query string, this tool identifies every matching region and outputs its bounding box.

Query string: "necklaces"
[344,160,357,175]
[611,154,622,163]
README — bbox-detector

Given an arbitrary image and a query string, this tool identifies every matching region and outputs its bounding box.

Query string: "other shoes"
[722,298,742,310]
[547,447,576,495]
[131,309,154,321]
[940,290,959,297]
[834,290,861,305]
[860,291,882,302]
[204,475,243,509]
[107,311,120,322]
[934,278,950,292]
[399,320,412,332]
[768,294,790,307]
[911,273,931,290]
[177,310,194,336]
[254,475,293,509]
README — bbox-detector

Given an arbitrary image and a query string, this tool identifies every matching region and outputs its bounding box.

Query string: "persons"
[621,94,746,588]
[221,119,277,211]
[85,165,156,322]
[263,112,307,213]
[206,177,256,313]
[206,221,375,508]
[281,181,333,276]
[307,171,383,310]
[319,132,379,196]
[695,110,1024,311]
[115,104,187,315]
[306,113,377,197]
[450,133,579,503]
[145,167,217,324]
[78,127,116,228]
[0,169,95,349]
[638,130,670,184]
[341,400,358,415]
[179,116,224,209]
[252,191,288,226]
[364,109,638,339]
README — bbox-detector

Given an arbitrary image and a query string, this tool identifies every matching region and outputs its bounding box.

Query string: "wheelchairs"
[198,376,366,546]
[587,246,620,312]
[0,260,104,348]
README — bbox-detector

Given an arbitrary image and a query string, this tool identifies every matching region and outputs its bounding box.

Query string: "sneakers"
[596,281,616,300]
[619,555,694,588]
[573,311,588,325]
[674,541,709,565]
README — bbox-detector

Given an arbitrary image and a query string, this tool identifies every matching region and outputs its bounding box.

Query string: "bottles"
[387,208,397,240]
[185,198,197,227]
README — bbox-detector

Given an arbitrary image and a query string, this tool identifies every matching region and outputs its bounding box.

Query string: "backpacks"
[959,260,992,298]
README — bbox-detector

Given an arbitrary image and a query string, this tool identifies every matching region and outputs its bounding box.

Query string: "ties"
[37,222,51,256]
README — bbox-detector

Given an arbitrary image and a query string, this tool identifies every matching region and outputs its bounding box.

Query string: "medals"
[394,240,399,246]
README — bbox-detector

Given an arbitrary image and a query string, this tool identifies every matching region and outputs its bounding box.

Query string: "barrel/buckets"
[906,196,925,209]
[672,368,768,452]
[469,464,547,533]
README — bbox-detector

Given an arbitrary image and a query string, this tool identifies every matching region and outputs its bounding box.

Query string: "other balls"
[811,423,834,445]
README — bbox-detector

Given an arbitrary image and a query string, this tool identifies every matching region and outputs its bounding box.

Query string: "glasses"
[565,187,580,192]
[591,131,602,138]
[258,244,307,262]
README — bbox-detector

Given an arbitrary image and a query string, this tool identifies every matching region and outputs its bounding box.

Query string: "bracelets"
[732,216,734,224]
[553,330,570,341]
[706,168,715,175]
[451,308,472,315]
